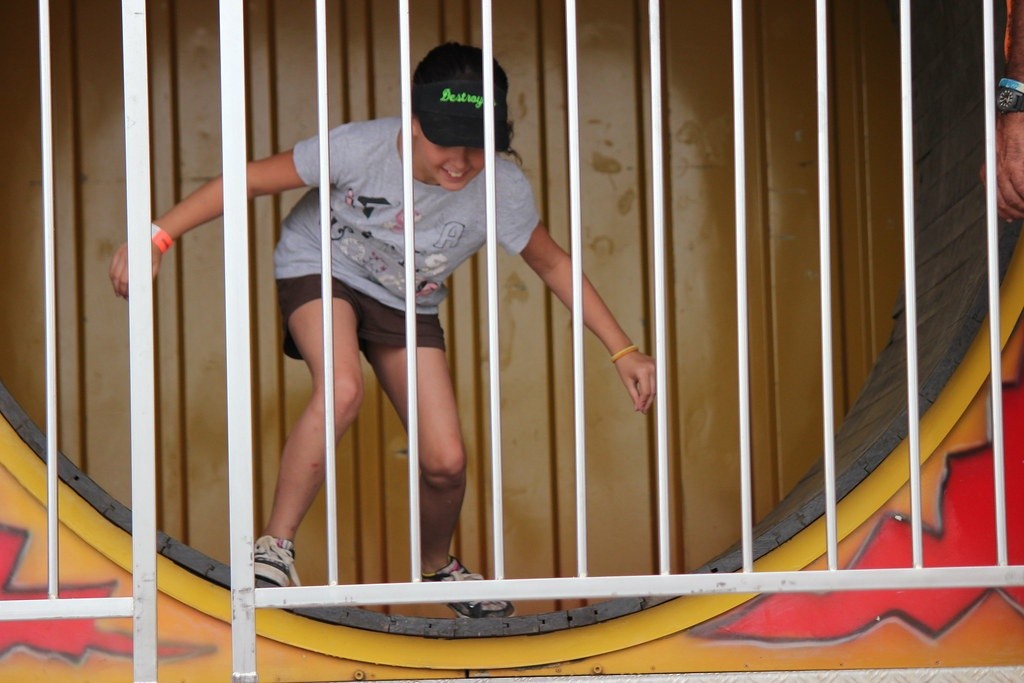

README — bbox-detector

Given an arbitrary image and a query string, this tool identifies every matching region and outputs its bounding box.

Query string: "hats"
[412,42,509,152]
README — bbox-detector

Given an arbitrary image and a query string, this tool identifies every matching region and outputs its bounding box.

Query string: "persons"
[108,40,656,618]
[978,0,1024,224]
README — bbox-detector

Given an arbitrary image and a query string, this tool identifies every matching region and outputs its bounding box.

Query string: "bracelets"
[610,343,639,364]
[997,77,1024,94]
[151,222,173,254]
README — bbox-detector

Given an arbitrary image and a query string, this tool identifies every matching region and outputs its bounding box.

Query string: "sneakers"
[254,534,301,588]
[422,557,516,618]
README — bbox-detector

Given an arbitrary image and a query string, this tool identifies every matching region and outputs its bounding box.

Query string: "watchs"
[996,87,1024,116]
